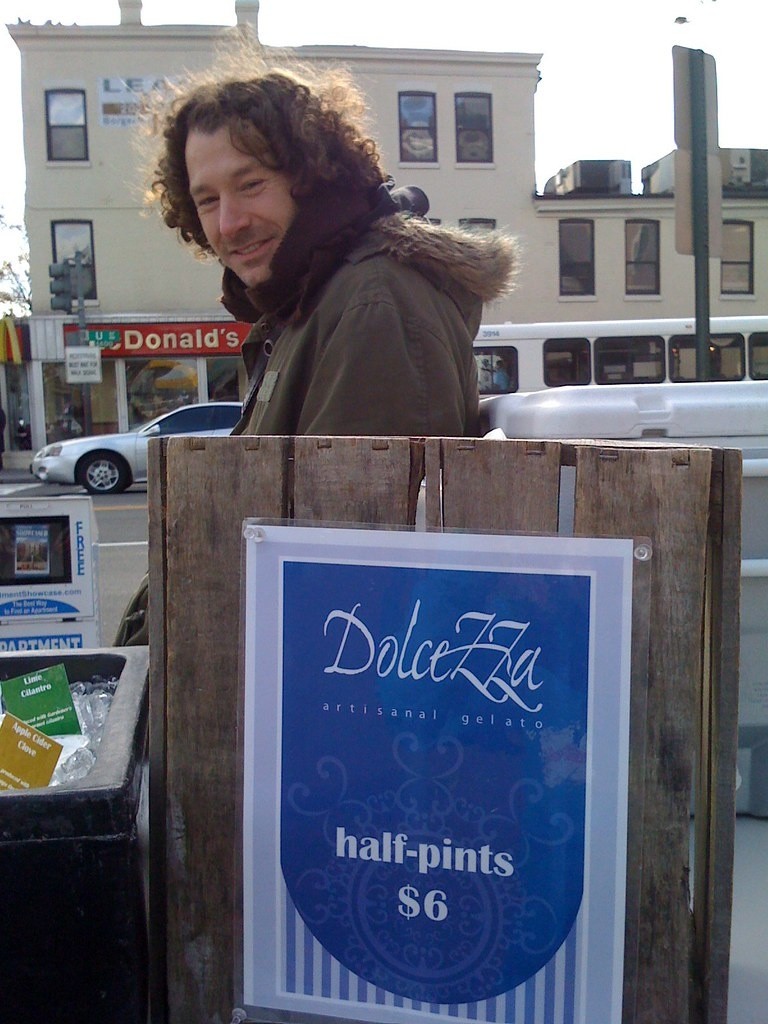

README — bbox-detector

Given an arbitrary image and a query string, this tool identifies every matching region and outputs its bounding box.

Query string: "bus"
[472,316,768,403]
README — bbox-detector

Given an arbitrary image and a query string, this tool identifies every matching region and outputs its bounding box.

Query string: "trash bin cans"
[0,643,163,1024]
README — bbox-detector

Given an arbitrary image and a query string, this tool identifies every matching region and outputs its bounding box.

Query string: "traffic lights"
[49,260,73,314]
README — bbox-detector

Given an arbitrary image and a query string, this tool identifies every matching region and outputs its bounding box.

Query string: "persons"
[110,70,475,717]
[486,360,509,393]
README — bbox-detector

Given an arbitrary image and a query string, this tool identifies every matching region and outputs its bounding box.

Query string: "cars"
[29,400,246,495]
